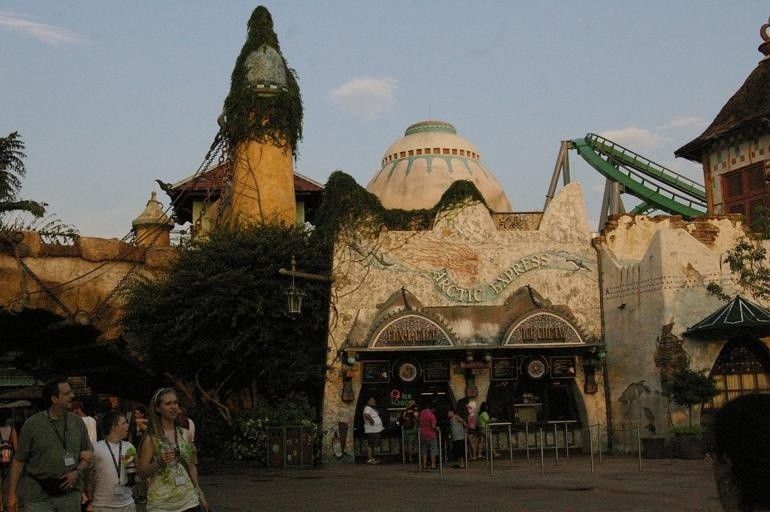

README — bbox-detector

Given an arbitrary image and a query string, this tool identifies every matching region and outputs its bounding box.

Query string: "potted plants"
[663,363,721,460]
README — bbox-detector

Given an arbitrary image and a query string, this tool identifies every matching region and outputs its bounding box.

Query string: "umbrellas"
[679,294,770,341]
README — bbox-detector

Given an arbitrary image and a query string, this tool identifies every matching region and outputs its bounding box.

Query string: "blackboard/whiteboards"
[491,358,518,381]
[423,359,450,382]
[550,356,578,379]
[361,360,391,384]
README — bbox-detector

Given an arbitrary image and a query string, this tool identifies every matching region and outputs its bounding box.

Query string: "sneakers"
[367,459,380,465]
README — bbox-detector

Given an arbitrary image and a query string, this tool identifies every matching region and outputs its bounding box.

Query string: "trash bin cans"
[267,425,313,467]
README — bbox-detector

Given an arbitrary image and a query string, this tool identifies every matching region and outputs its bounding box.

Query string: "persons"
[361,396,386,466]
[401,399,417,464]
[412,399,503,471]
[0,373,214,512]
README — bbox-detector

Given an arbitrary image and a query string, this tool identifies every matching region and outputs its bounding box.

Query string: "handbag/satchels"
[39,477,66,497]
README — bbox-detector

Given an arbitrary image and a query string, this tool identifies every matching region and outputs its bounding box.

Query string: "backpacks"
[0,426,15,467]
[404,410,415,428]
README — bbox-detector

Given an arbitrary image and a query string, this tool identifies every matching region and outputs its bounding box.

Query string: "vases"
[641,438,666,459]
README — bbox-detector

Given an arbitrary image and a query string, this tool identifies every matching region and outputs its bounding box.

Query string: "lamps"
[279,254,332,321]
